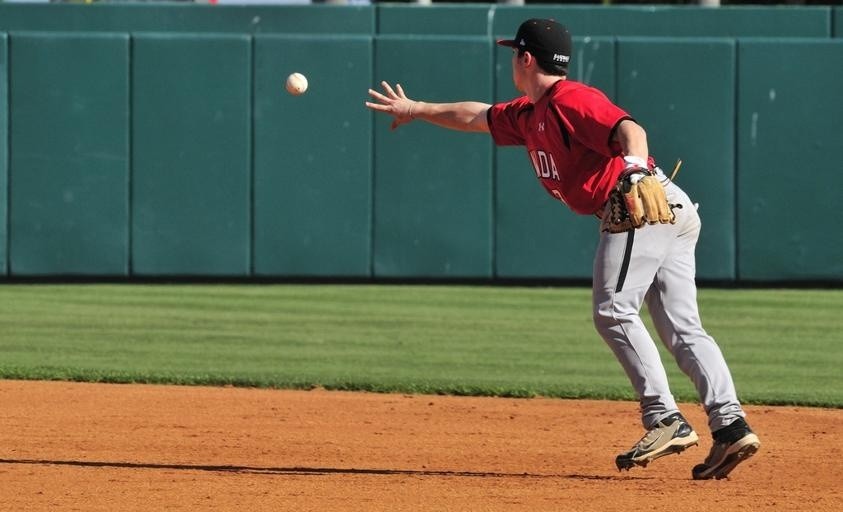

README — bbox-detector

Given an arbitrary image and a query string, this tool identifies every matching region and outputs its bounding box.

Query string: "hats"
[497,18,572,63]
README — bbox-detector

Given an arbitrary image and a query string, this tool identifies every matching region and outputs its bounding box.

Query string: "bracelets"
[406,96,419,119]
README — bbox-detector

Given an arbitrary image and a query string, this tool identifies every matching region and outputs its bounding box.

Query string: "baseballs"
[285,73,308,95]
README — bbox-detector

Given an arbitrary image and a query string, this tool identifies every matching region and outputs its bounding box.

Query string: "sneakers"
[616,414,699,469]
[692,419,760,479]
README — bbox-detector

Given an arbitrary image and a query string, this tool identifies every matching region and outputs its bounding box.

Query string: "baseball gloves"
[599,168,676,236]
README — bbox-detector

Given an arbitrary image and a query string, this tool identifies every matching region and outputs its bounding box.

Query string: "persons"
[364,17,761,479]
